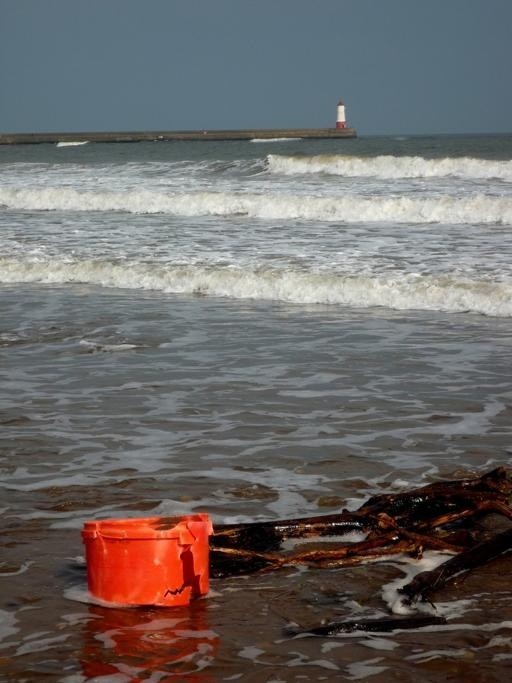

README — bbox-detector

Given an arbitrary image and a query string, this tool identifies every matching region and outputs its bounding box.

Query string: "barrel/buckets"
[81,512,214,610]
